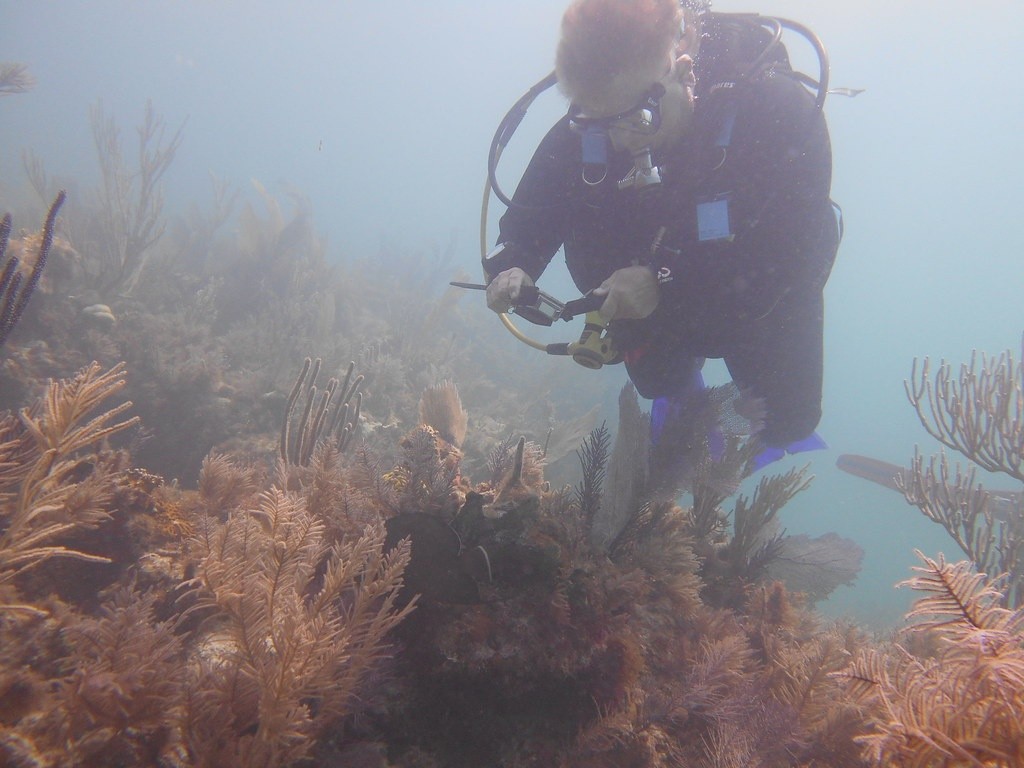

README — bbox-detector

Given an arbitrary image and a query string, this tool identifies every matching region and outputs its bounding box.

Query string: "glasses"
[568,81,667,136]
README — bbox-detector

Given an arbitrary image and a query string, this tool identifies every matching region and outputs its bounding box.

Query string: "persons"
[481,0,840,445]
